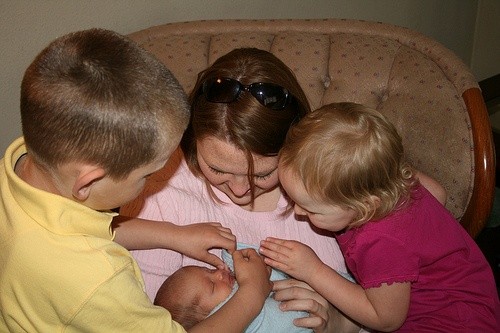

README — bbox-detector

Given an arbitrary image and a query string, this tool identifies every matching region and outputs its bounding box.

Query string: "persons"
[153,243,357,333]
[0,27,275,333]
[118,48,384,333]
[259,101,500,333]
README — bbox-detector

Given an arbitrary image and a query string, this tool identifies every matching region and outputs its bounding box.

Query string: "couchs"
[118,19,495,240]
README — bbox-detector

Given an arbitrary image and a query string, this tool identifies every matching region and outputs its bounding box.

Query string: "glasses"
[186,75,305,122]
